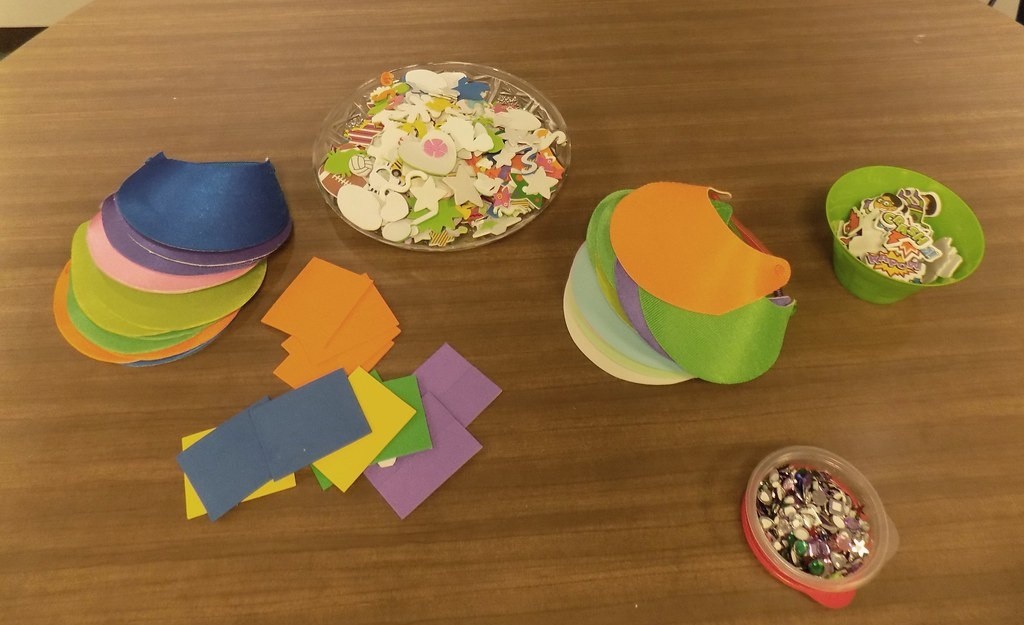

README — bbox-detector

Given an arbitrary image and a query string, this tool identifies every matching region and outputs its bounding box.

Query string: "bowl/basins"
[825,165,985,303]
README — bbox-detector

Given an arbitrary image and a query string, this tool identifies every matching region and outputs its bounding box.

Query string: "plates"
[311,61,572,252]
